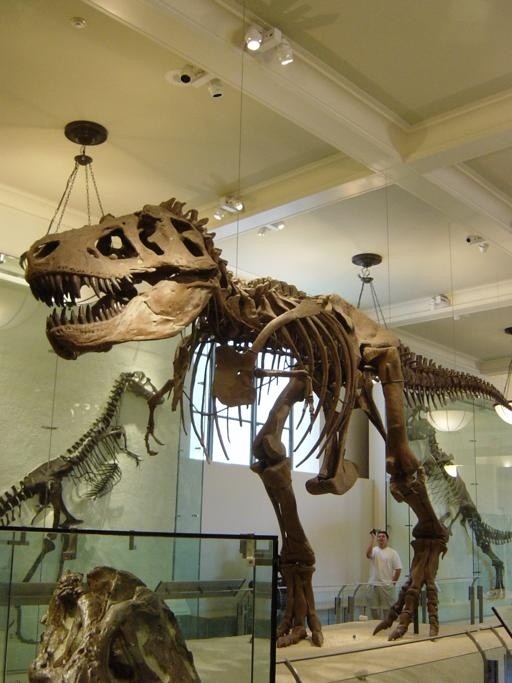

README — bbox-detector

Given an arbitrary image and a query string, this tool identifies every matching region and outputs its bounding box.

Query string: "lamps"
[425,407,474,432]
[493,359,511,425]
[242,22,294,66]
[179,62,224,100]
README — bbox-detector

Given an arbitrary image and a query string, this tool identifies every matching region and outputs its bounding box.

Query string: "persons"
[366,528,403,622]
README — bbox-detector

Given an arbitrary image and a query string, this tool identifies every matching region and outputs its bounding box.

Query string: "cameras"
[369,529,376,536]
